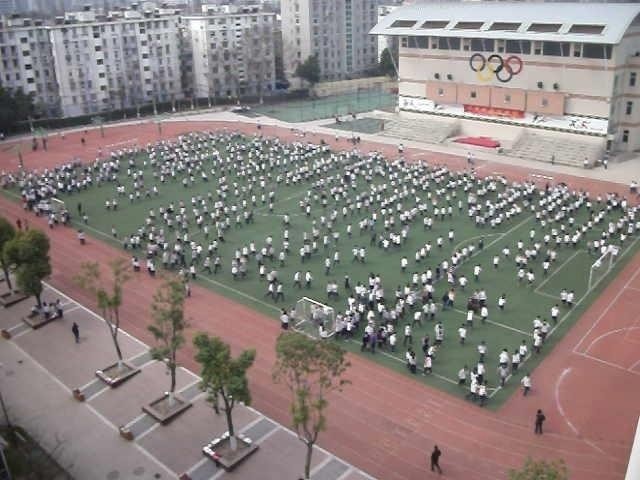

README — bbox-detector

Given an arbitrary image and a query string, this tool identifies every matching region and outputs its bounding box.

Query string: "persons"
[8,117,640,408]
[535,410,545,435]
[430,445,442,474]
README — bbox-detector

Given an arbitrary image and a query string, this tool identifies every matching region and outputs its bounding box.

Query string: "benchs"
[378,115,461,145]
[504,128,606,170]
